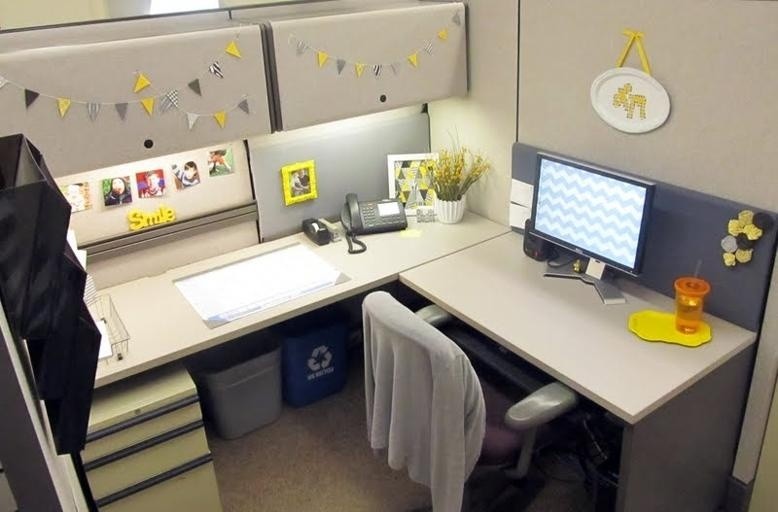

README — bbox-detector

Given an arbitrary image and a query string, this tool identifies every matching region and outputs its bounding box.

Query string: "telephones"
[340,193,407,236]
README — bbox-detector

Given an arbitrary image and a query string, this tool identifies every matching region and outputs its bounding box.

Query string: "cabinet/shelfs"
[68,362,224,512]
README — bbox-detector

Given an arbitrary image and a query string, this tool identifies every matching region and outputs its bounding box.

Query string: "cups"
[674,278,711,335]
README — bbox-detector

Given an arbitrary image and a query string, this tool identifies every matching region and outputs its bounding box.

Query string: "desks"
[398,229,756,425]
[62,199,513,394]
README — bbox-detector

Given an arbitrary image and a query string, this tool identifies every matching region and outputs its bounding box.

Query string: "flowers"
[421,124,491,200]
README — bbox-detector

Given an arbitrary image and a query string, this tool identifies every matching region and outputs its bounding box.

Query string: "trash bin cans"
[197,340,283,440]
[580,413,623,512]
[274,310,349,407]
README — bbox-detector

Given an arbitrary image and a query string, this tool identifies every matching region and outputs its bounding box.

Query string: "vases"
[433,192,468,223]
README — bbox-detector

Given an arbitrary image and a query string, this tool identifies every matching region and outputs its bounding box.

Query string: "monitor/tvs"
[528,151,658,305]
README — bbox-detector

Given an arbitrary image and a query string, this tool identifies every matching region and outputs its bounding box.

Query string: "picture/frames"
[386,152,442,217]
[588,65,671,135]
[280,160,318,207]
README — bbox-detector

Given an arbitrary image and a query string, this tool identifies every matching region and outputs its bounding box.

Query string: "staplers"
[318,218,341,242]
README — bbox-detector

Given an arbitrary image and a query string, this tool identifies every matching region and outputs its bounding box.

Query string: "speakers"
[523,219,554,260]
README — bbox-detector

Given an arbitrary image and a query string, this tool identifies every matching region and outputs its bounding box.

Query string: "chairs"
[361,290,578,512]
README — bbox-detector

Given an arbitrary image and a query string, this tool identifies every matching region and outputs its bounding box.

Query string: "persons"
[171,162,200,189]
[207,150,234,176]
[138,170,166,198]
[104,178,132,206]
[290,169,310,196]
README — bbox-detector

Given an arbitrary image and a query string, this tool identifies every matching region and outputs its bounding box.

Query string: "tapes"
[312,222,319,233]
